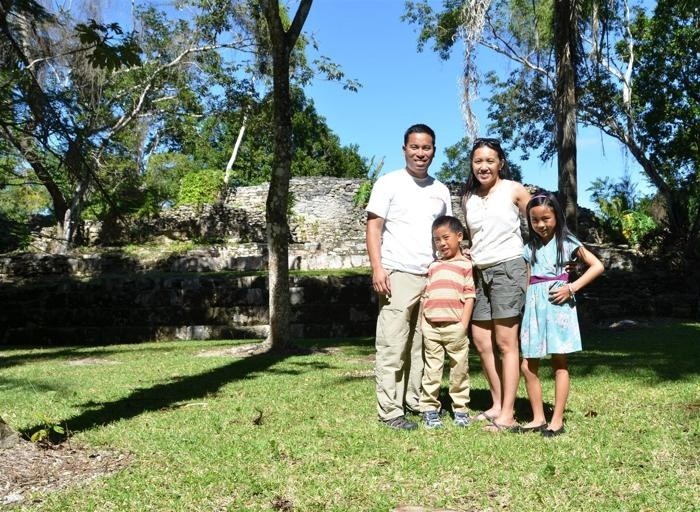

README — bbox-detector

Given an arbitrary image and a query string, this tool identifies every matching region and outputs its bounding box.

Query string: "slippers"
[473,412,517,432]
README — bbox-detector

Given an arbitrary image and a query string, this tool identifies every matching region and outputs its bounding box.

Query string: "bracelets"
[567,282,577,303]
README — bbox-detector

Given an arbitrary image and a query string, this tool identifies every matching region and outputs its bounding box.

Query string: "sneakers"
[405,407,446,417]
[418,411,445,435]
[384,416,416,431]
[453,412,470,426]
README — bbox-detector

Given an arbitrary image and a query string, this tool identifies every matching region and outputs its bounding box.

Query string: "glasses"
[474,138,500,147]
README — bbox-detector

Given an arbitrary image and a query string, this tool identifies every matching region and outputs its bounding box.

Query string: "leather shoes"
[510,424,548,434]
[540,426,565,437]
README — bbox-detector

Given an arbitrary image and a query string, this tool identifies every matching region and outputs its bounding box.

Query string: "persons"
[518,191,606,437]
[363,124,455,430]
[460,137,535,434]
[419,215,478,430]
